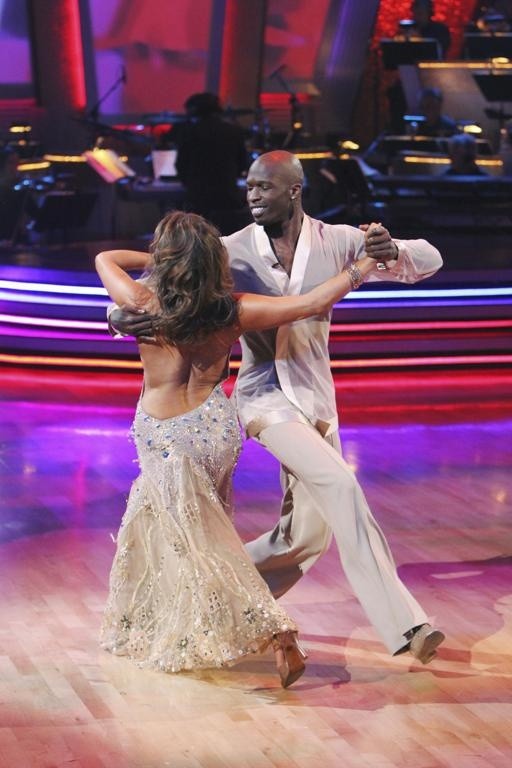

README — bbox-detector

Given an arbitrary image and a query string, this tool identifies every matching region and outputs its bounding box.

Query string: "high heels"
[273,629,309,689]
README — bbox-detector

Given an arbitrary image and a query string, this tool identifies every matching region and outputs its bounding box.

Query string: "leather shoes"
[408,624,446,665]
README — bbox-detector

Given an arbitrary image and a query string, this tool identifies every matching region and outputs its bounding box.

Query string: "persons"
[384,0,452,136]
[93,208,385,689]
[404,84,461,138]
[106,147,449,667]
[156,90,260,235]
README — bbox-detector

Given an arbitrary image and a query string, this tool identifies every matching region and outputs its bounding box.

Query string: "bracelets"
[346,259,364,290]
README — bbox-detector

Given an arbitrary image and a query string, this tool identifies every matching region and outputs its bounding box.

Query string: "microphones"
[269,63,286,81]
[119,63,126,83]
